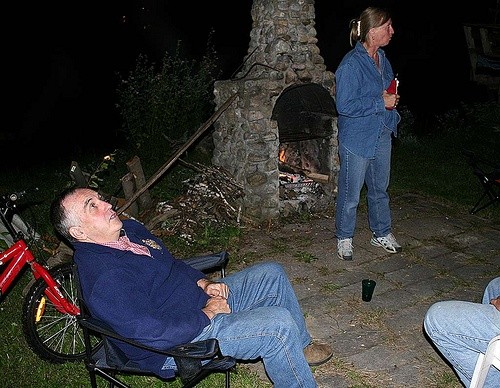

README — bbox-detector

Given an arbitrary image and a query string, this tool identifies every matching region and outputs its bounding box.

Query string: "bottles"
[385,73,399,111]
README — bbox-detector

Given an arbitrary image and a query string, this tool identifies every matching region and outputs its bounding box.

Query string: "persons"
[335,5,402,260]
[50,186,333,388]
[424,276,500,388]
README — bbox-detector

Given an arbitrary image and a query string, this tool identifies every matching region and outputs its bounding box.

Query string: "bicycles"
[0,188,107,366]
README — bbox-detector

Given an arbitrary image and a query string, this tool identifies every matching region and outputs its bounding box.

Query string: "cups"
[362,280,376,302]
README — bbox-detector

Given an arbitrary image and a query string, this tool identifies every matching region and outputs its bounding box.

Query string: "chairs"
[71,250,236,388]
[462,150,500,215]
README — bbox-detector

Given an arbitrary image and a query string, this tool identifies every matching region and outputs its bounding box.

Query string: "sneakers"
[303,341,332,366]
[337,238,353,261]
[370,233,402,253]
